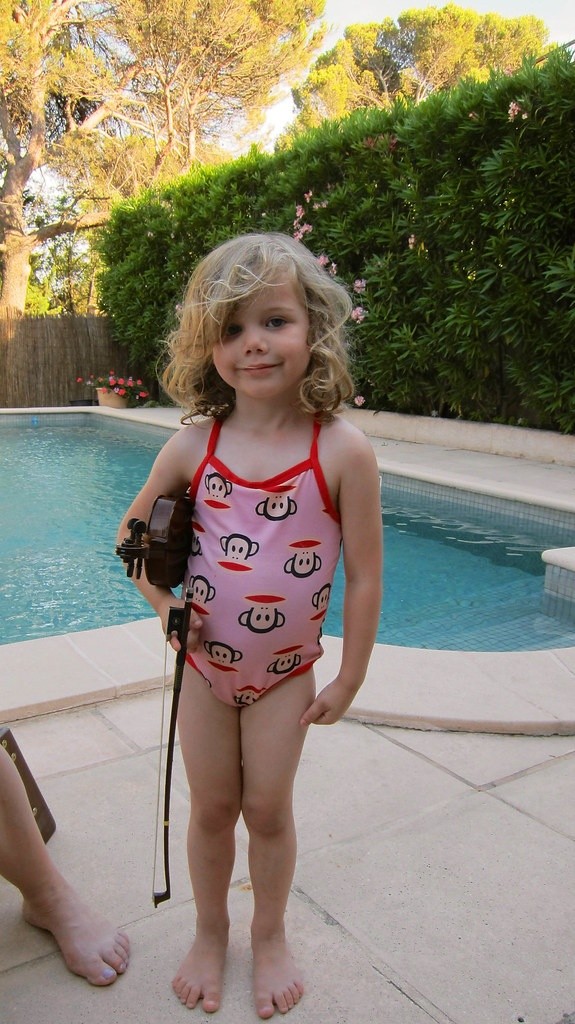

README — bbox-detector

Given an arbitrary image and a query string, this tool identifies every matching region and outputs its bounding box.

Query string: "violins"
[115,493,193,588]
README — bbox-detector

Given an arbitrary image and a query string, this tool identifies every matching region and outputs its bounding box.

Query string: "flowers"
[73,370,153,402]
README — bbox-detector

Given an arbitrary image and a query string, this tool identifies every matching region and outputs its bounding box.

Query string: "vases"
[95,388,129,411]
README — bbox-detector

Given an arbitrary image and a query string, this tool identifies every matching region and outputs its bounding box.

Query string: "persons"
[1,740,133,988]
[114,232,385,1019]
